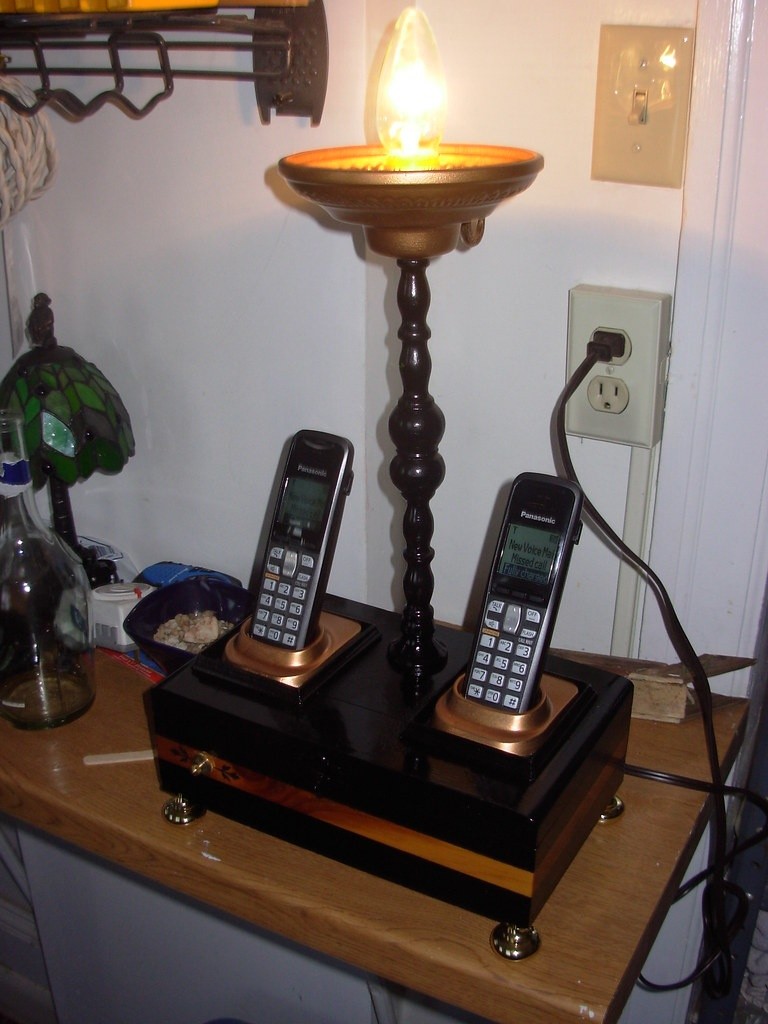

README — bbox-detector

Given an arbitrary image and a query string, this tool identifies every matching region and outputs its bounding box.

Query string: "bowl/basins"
[122,579,246,676]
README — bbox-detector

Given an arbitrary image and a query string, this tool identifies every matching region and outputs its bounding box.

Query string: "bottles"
[0,408,96,732]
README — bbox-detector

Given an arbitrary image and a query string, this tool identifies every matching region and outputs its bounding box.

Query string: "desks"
[0,649,753,1024]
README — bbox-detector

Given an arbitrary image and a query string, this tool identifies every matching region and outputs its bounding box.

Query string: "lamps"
[0,292,137,588]
[277,8,543,751]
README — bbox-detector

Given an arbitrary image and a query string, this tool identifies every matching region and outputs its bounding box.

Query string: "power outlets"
[564,286,672,448]
